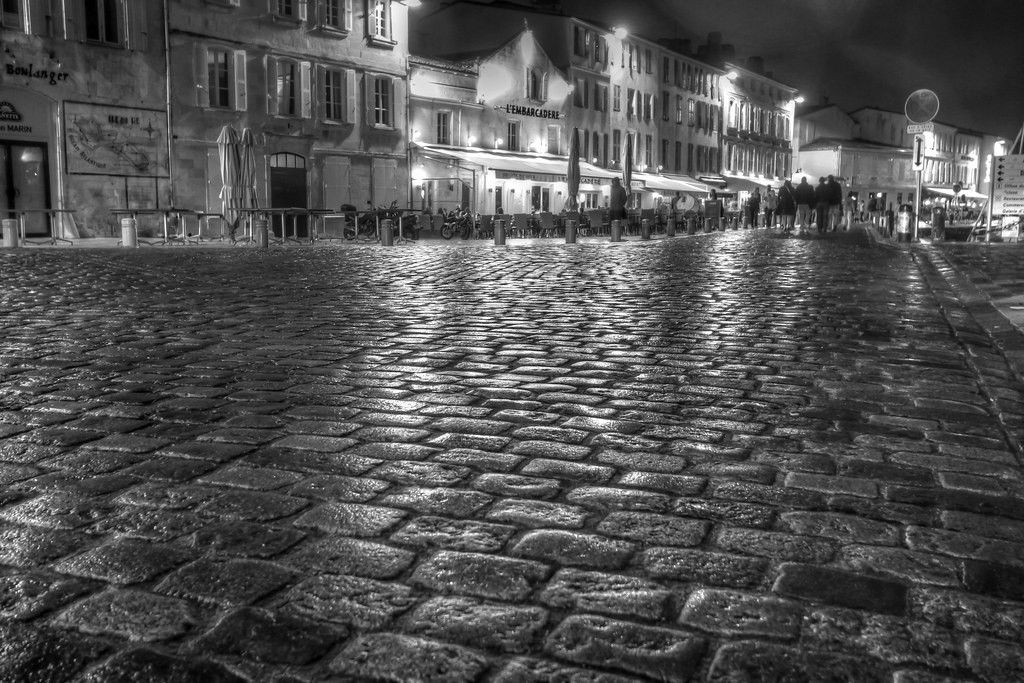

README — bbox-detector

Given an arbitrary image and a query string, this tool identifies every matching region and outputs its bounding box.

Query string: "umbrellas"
[621,130,633,206]
[564,127,580,212]
[217,124,260,241]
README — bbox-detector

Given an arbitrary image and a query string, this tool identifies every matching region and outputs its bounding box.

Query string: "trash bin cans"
[668,219,675,236]
[642,219,651,240]
[494,220,506,245]
[2,219,19,248]
[687,219,695,235]
[121,218,139,247]
[732,217,738,230]
[719,217,725,231]
[565,220,576,243]
[381,219,393,246]
[611,220,621,242]
[705,218,711,233]
[255,220,268,247]
[742,216,748,228]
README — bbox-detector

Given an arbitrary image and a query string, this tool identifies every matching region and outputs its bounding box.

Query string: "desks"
[291,208,335,246]
[108,208,156,246]
[150,208,190,246]
[383,209,423,245]
[367,210,391,245]
[0,208,44,246]
[35,208,76,246]
[260,207,306,246]
[181,212,221,246]
[336,210,371,244]
[168,210,204,246]
[228,208,262,247]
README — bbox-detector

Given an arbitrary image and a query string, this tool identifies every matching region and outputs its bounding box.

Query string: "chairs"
[674,209,685,234]
[540,212,560,238]
[588,210,611,237]
[513,213,534,238]
[499,213,516,239]
[634,208,655,236]
[656,210,669,234]
[565,212,589,237]
[476,214,493,240]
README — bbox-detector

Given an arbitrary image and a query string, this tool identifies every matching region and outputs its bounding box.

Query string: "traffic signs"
[986,153,1024,215]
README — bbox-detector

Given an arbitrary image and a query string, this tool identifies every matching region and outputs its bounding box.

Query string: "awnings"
[608,171,801,198]
[408,140,646,189]
[924,188,988,199]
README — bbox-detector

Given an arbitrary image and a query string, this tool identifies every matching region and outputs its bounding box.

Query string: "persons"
[422,205,471,222]
[474,205,584,239]
[858,195,968,225]
[608,175,856,237]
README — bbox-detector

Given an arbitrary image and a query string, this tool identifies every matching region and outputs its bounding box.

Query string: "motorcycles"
[341,201,424,239]
[439,206,472,241]
